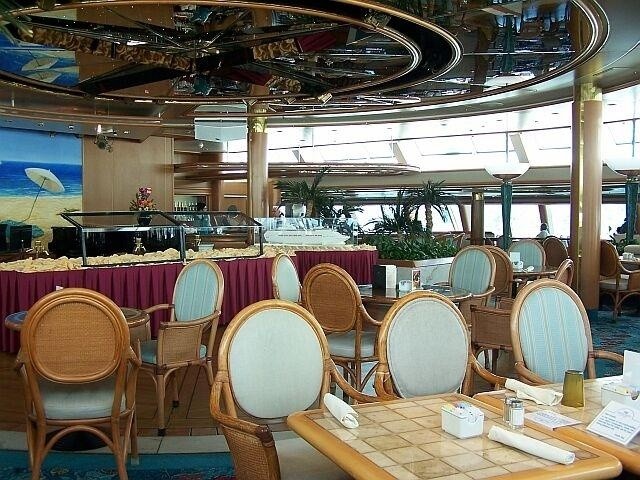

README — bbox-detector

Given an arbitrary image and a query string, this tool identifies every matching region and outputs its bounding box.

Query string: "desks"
[0,251,379,354]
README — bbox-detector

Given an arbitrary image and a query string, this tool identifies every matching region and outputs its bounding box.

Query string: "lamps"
[242,98,258,106]
[317,92,332,106]
[94,123,117,154]
[483,160,530,249]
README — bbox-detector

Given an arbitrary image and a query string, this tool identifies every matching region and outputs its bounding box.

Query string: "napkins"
[505,379,564,405]
[323,393,359,428]
[487,427,576,465]
[601,350,640,406]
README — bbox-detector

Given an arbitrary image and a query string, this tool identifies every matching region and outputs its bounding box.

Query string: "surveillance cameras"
[96,133,107,149]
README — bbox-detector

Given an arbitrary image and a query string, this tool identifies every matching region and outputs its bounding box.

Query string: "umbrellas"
[29,70,61,84]
[21,50,59,72]
[24,167,65,218]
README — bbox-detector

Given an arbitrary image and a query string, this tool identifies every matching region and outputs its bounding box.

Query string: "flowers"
[129,186,159,211]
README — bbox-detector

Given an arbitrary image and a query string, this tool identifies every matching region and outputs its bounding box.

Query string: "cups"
[399,280,412,292]
[560,369,585,407]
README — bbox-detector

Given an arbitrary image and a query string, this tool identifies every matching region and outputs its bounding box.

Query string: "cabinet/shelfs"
[163,211,264,260]
[255,217,359,251]
[56,211,183,267]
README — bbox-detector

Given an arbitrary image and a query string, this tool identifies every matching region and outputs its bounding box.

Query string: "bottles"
[504,398,526,428]
[173,200,198,211]
[623,252,633,259]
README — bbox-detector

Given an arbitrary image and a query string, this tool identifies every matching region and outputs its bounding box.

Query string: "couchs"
[624,245,640,258]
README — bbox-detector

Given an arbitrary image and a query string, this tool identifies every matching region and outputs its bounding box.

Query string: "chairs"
[485,232,504,246]
[470,259,574,389]
[510,278,623,386]
[373,290,517,401]
[508,237,569,266]
[140,259,224,437]
[600,240,640,323]
[481,245,512,298]
[271,253,302,305]
[14,288,138,479]
[210,299,389,480]
[302,263,383,404]
[434,245,497,369]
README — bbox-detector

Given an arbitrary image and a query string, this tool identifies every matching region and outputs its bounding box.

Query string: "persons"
[536,223,550,238]
[195,203,214,234]
[221,204,245,226]
